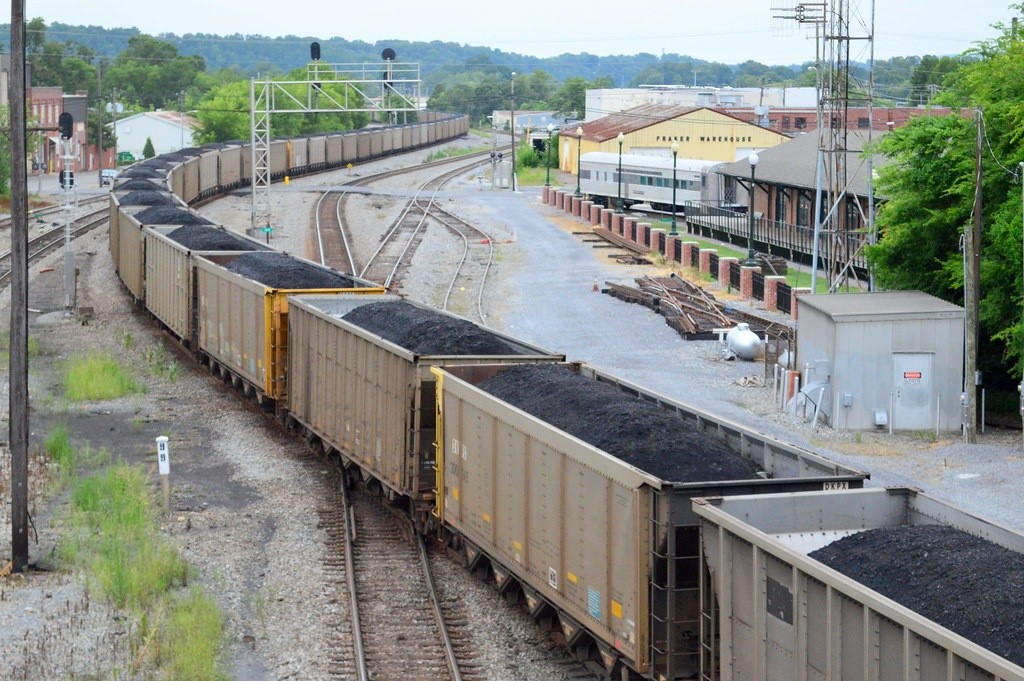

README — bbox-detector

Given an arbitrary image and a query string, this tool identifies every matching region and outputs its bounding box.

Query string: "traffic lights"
[490,153,495,158]
[497,153,503,158]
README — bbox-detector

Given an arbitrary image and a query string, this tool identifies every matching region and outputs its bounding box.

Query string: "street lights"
[180,110,199,149]
[669,138,680,236]
[573,126,584,198]
[104,91,123,169]
[615,131,625,214]
[746,152,759,266]
[544,123,554,186]
[509,71,517,191]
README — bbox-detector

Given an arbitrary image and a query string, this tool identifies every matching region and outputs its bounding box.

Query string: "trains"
[107,104,1024,681]
[575,150,749,216]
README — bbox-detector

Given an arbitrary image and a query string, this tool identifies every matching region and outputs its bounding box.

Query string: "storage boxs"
[105,109,1024,681]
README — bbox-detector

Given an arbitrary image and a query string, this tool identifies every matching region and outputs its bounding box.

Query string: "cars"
[118,152,136,166]
[99,169,118,185]
